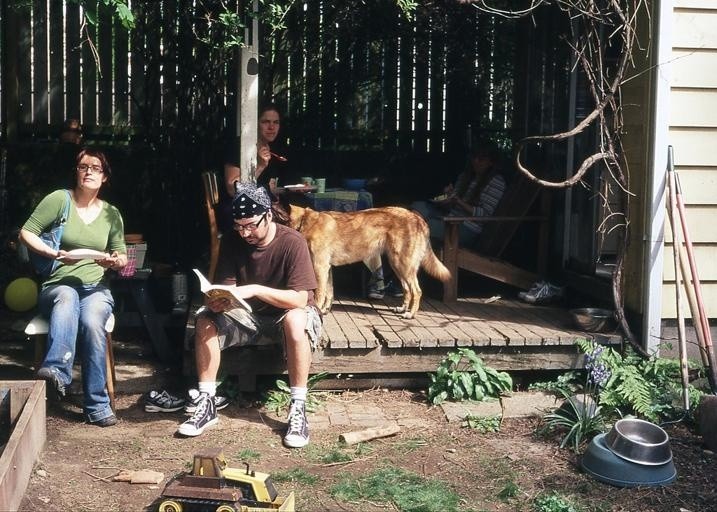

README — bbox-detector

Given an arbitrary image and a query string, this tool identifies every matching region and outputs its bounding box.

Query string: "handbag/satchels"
[18,224,63,283]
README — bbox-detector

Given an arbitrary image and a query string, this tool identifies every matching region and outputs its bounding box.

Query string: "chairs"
[432,160,556,302]
[24,313,116,415]
[202,170,244,286]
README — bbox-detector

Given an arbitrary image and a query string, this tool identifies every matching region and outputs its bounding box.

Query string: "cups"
[315,179,325,193]
[303,177,313,184]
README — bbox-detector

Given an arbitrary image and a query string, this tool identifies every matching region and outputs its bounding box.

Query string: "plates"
[285,183,317,194]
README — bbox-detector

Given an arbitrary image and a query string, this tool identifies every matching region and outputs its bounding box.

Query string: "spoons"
[270,150,288,161]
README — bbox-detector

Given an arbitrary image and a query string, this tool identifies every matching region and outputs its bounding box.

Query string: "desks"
[105,268,174,368]
[305,188,386,299]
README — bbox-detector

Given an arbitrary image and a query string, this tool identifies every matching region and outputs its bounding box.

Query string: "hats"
[230,181,272,221]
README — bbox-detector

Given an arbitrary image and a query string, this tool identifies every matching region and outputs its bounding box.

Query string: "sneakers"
[37,367,63,405]
[367,283,385,300]
[524,283,552,305]
[283,399,311,447]
[177,391,219,436]
[517,279,545,300]
[93,415,118,427]
[388,282,403,298]
[184,387,232,412]
[143,389,184,413]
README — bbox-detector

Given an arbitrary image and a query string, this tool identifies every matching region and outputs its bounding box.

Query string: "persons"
[365,136,507,300]
[20,148,129,428]
[216,103,286,238]
[175,180,324,448]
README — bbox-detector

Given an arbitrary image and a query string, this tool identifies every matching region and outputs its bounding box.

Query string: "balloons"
[4,277,38,312]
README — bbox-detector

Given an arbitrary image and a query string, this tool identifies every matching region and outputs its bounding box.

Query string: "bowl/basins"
[604,419,673,467]
[582,432,677,488]
[571,308,622,331]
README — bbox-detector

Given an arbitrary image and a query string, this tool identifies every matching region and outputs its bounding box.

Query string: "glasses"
[75,163,105,172]
[230,212,267,232]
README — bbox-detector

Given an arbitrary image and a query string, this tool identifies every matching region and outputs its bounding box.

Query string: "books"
[190,267,253,313]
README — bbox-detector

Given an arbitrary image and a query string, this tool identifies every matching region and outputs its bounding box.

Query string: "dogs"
[271,202,451,319]
